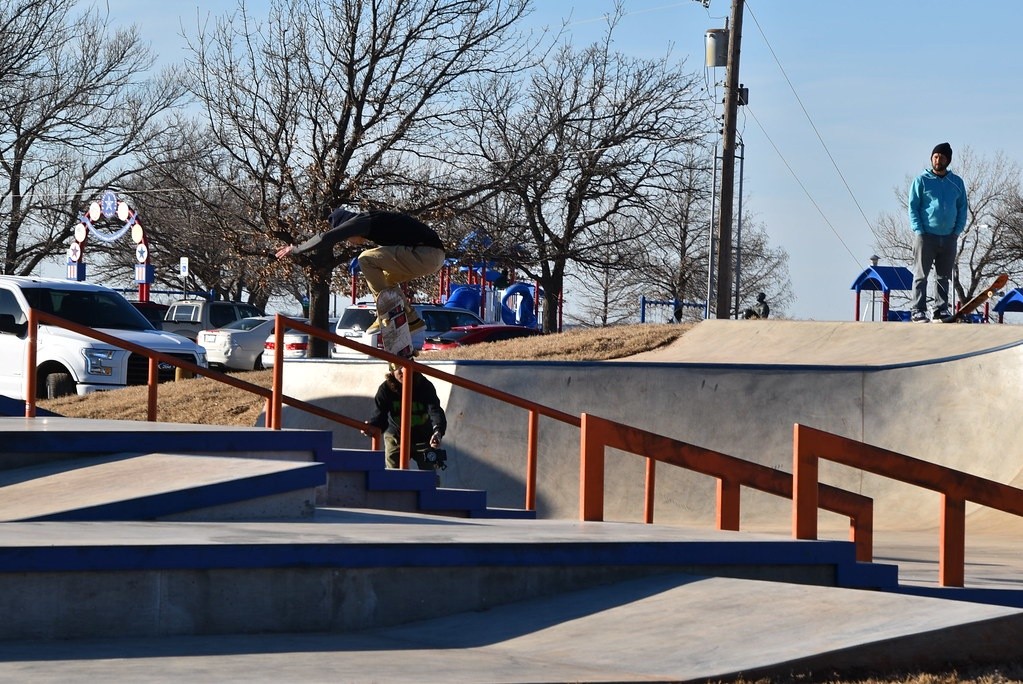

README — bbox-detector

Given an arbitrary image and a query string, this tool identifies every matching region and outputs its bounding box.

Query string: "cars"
[128,299,170,334]
[423,325,547,354]
[0,274,208,403]
[165,300,266,340]
[197,316,309,370]
[261,317,338,370]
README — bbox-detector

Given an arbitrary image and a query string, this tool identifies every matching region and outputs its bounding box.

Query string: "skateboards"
[376,287,419,370]
[943,272,1008,323]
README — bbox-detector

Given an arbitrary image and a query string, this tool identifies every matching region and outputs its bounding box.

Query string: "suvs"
[331,300,498,360]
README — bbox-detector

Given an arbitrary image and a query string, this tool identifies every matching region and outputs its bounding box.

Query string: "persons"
[360,361,447,480]
[277,203,447,338]
[907,141,969,324]
[743,290,769,319]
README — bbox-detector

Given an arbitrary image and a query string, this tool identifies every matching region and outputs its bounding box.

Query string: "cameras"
[423,447,447,464]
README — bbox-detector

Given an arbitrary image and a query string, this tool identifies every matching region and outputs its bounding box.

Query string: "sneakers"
[367,316,427,335]
[933,312,954,322]
[911,312,930,323]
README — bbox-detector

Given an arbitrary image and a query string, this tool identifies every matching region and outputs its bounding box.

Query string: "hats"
[932,143,952,162]
[329,204,363,230]
